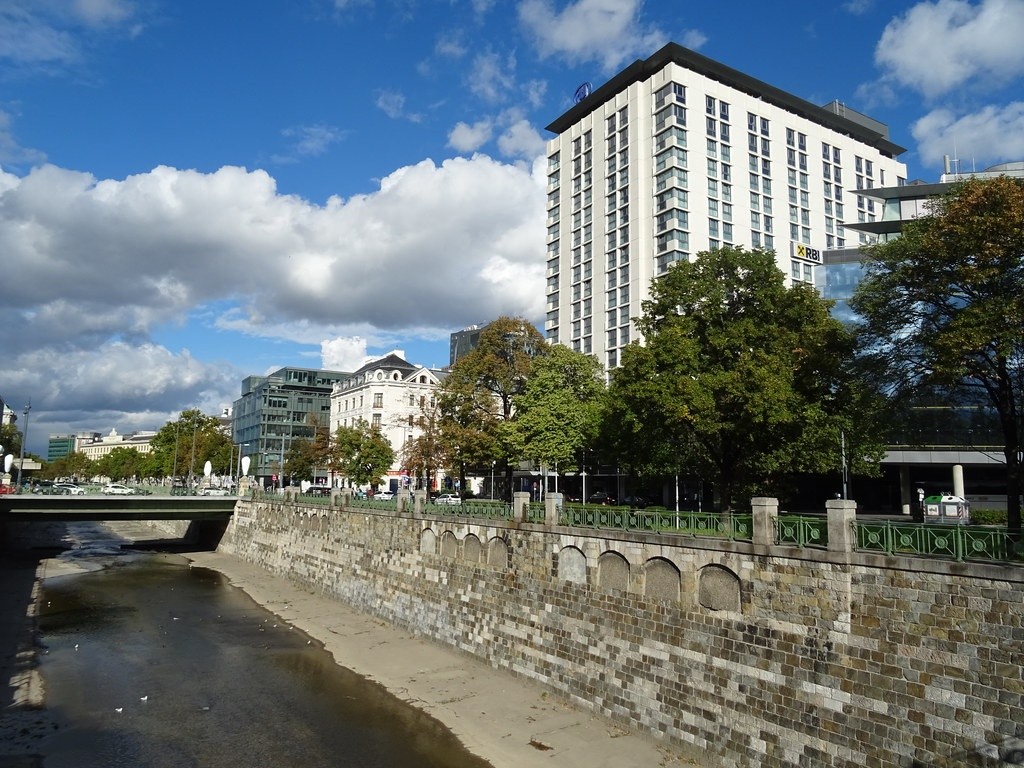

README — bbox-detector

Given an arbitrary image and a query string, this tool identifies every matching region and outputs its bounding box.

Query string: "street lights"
[230,445,239,493]
[16,405,31,495]
[492,460,496,501]
[236,444,250,495]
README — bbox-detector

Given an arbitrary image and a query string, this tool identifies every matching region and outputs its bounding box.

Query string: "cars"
[426,492,439,504]
[30,481,71,496]
[56,484,86,495]
[434,494,461,505]
[306,487,331,496]
[374,491,393,501]
[101,484,136,495]
[621,495,649,509]
[587,491,616,505]
[0,484,17,494]
[172,478,183,487]
[199,486,230,496]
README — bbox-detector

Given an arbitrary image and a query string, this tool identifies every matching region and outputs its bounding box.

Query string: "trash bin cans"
[923,495,970,525]
